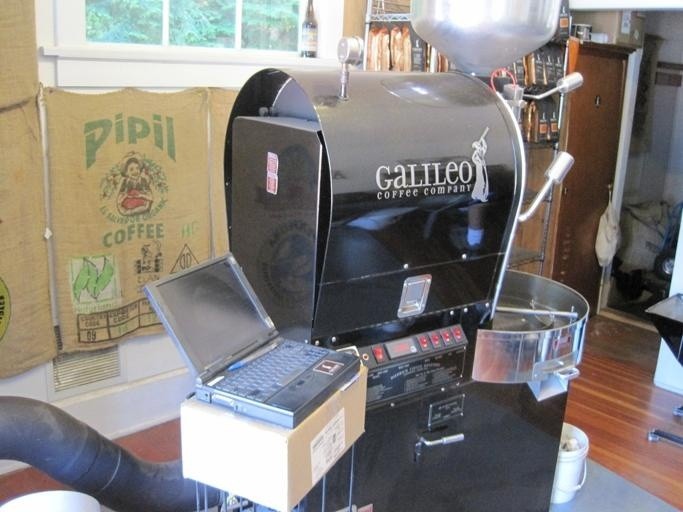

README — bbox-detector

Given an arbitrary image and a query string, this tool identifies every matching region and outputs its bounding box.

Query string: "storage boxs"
[572,9,648,48]
[180,364,368,512]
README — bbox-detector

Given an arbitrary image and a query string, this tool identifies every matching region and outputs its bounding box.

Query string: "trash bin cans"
[551,422,590,504]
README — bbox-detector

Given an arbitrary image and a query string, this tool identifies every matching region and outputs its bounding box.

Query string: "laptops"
[144,252,361,429]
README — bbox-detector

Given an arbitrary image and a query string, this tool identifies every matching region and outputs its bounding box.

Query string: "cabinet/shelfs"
[510,38,634,291]
[363,0,456,73]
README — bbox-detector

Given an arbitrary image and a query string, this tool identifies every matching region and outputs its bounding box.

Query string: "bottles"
[558,5,569,39]
[300,1,318,59]
